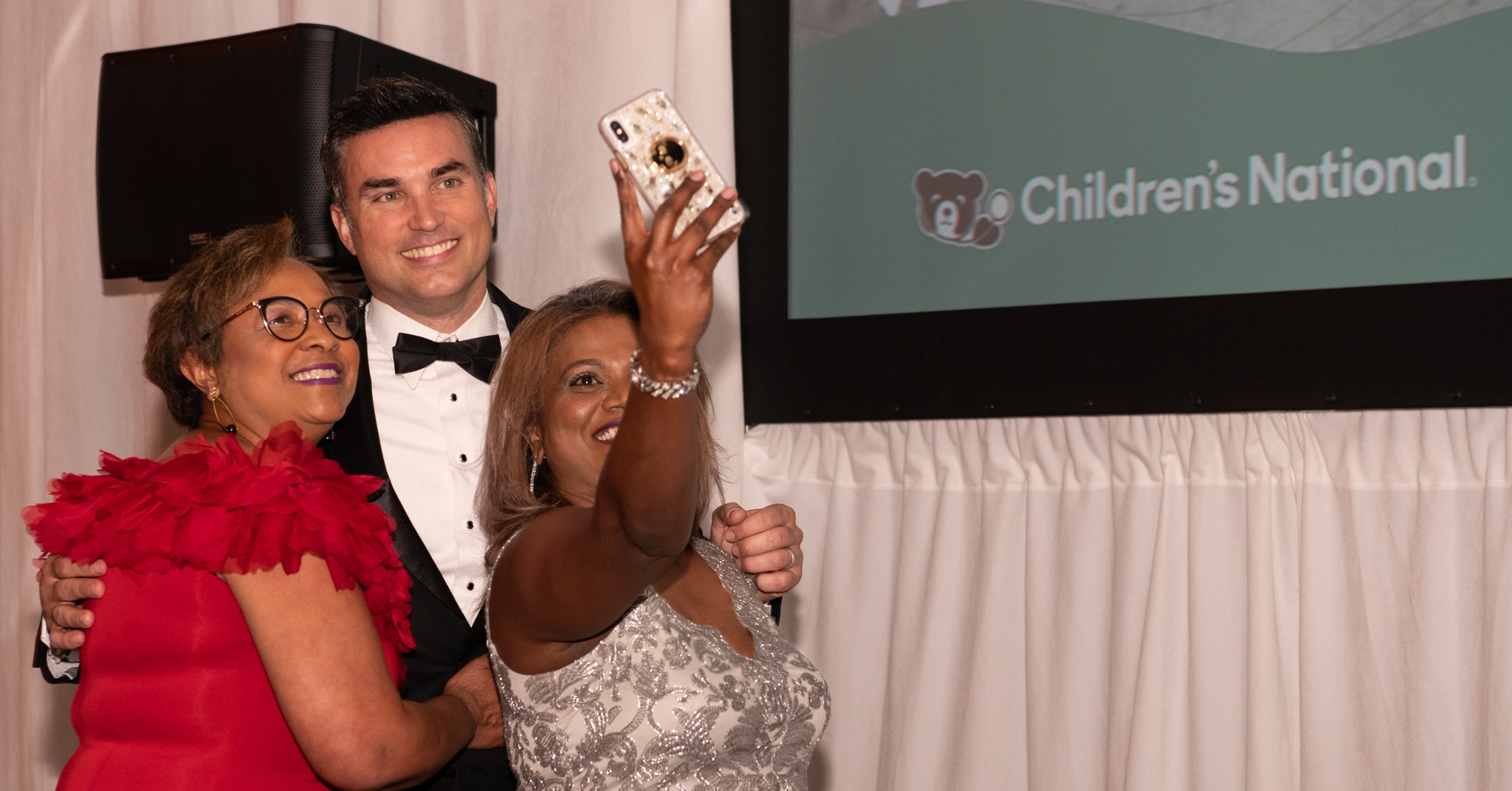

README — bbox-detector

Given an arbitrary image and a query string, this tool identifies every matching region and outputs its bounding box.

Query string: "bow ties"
[390,332,502,387]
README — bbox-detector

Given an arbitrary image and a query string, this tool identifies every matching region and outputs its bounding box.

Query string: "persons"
[474,154,832,788]
[26,72,801,787]
[18,217,509,791]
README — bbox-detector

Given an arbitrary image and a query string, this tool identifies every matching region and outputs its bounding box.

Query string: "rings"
[782,548,796,570]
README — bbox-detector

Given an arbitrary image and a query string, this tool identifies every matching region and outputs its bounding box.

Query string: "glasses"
[189,295,369,345]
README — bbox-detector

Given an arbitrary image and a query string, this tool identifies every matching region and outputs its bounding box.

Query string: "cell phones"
[598,86,748,254]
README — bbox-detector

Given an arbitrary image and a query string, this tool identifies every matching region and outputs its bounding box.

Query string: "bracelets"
[627,350,704,403]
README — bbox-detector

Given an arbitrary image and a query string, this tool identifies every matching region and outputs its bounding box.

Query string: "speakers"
[92,23,503,292]
